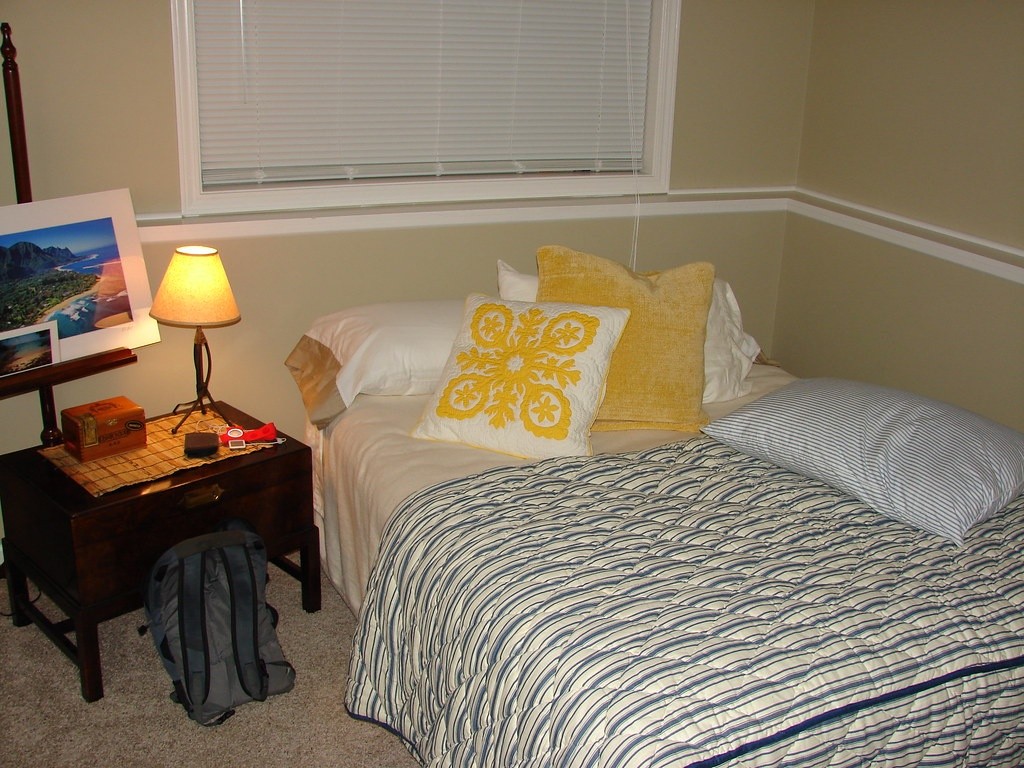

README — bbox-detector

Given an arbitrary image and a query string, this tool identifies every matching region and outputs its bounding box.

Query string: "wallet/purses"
[184,431,219,456]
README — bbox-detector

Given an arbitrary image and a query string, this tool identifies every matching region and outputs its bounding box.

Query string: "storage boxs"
[60,396,148,465]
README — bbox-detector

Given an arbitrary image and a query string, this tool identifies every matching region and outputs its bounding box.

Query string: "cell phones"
[228,439,245,449]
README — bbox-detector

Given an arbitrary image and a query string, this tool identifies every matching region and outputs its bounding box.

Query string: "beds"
[284,295,1024,768]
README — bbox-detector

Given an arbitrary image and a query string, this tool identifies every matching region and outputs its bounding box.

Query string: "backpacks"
[139,531,296,726]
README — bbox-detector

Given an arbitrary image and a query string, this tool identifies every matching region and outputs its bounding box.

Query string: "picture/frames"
[0,188,162,379]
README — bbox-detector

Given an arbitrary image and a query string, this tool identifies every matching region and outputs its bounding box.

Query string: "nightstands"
[0,400,321,703]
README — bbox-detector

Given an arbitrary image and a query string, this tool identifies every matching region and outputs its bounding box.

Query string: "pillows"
[533,244,714,433]
[697,378,1024,548]
[284,300,465,430]
[405,290,632,459]
[495,259,763,406]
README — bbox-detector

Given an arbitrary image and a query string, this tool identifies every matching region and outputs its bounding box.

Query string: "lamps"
[148,247,244,434]
